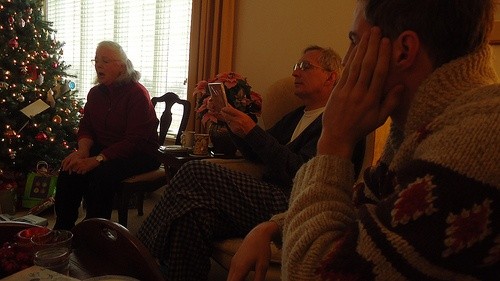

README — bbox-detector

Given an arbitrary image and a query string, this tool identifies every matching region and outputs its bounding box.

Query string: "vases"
[209,121,238,155]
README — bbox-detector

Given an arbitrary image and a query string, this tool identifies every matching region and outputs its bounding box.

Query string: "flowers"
[193,72,263,128]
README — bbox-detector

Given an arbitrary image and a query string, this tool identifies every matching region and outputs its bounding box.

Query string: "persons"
[51,40,162,230]
[134,44,366,281]
[229,0,500,281]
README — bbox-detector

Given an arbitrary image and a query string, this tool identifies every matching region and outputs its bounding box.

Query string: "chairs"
[116,93,191,228]
[211,130,376,281]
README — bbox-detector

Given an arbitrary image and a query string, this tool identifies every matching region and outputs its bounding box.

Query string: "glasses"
[94,59,120,66]
[292,62,323,71]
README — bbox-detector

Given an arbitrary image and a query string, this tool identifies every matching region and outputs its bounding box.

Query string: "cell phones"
[208,82,228,112]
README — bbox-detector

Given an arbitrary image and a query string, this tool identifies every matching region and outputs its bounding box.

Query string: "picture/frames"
[208,82,228,113]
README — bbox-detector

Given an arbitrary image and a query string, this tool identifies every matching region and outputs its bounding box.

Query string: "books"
[208,82,228,112]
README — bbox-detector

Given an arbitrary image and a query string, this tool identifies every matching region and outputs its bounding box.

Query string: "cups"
[180,131,196,150]
[193,134,209,155]
[30,229,74,276]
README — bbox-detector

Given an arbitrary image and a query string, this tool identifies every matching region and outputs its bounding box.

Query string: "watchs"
[96,155,104,166]
